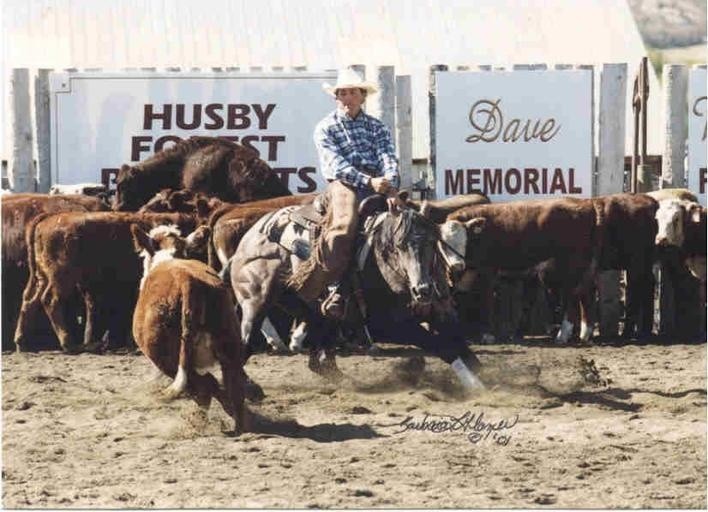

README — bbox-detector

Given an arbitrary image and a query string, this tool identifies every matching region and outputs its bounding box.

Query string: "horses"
[218,199,491,400]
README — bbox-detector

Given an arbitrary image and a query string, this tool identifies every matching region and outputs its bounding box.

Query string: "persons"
[315,69,404,318]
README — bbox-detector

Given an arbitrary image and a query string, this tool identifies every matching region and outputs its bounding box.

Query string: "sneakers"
[326,291,348,317]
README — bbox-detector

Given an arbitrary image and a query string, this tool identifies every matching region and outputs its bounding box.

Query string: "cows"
[110,137,292,212]
[1,184,707,354]
[131,223,255,433]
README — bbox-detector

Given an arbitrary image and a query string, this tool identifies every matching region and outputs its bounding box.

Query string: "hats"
[318,68,377,99]
